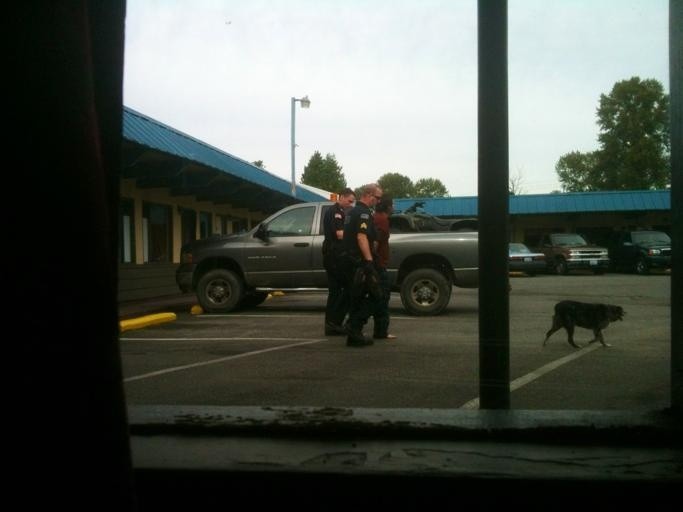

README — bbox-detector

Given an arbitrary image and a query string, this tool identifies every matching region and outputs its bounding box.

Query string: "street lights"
[289,93,310,194]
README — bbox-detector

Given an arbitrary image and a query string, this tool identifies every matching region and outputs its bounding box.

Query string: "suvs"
[534,230,612,277]
[606,229,671,275]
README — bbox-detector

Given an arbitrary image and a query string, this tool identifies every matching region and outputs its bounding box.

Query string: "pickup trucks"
[173,193,477,319]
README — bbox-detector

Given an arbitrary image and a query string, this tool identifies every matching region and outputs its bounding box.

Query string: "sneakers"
[346,334,372,347]
[325,326,345,336]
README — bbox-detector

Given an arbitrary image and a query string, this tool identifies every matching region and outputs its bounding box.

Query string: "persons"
[321,182,397,348]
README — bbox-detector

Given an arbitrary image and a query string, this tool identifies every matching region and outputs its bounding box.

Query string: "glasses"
[366,191,381,200]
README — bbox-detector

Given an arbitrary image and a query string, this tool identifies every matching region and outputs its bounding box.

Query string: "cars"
[507,241,547,278]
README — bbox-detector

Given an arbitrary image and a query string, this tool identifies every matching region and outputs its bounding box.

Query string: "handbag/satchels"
[351,263,384,317]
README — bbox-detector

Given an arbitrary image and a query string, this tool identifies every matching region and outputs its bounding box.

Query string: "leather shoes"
[387,334,397,339]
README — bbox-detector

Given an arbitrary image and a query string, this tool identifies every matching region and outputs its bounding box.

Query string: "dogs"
[543,300,627,348]
